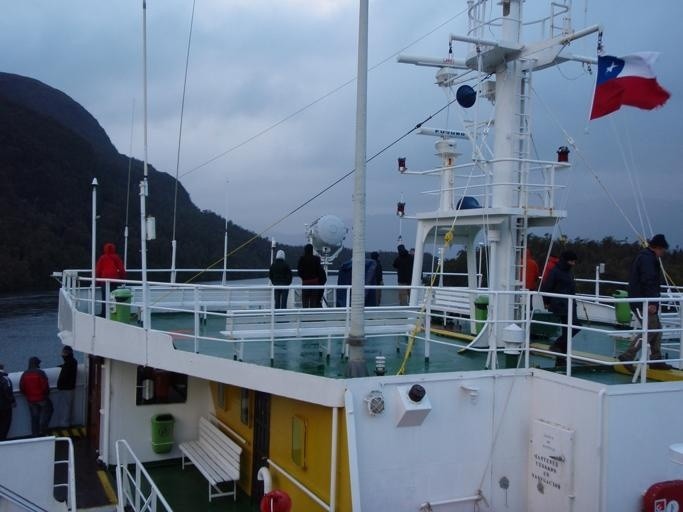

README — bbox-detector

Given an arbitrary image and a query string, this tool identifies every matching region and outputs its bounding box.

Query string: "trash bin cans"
[150,412,176,454]
[612,289,631,323]
[109,289,133,324]
[473,295,488,336]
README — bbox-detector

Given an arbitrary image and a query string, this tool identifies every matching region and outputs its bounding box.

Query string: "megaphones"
[456,84,483,109]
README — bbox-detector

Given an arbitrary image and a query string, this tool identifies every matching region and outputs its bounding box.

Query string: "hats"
[652,235,668,249]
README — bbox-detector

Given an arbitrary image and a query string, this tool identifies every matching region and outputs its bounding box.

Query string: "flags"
[588,52,671,120]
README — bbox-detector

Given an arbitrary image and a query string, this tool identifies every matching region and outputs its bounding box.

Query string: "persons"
[297,244,320,307]
[96,243,128,316]
[19,357,54,437]
[371,252,383,304]
[317,264,325,308]
[392,244,413,298]
[521,249,578,367]
[0,365,17,441]
[618,235,673,373]
[269,249,292,309]
[56,345,77,428]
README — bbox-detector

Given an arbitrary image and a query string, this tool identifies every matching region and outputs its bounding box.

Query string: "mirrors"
[288,413,309,472]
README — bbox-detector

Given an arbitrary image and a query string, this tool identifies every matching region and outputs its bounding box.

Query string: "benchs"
[178,416,244,504]
[420,286,527,328]
[220,306,418,363]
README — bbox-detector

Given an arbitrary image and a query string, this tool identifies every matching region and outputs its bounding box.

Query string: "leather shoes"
[651,363,672,370]
[619,356,635,373]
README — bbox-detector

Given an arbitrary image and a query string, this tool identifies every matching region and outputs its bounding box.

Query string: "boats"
[1,0,682,511]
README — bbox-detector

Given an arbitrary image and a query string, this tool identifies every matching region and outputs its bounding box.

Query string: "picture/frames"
[217,383,228,412]
[240,389,253,429]
